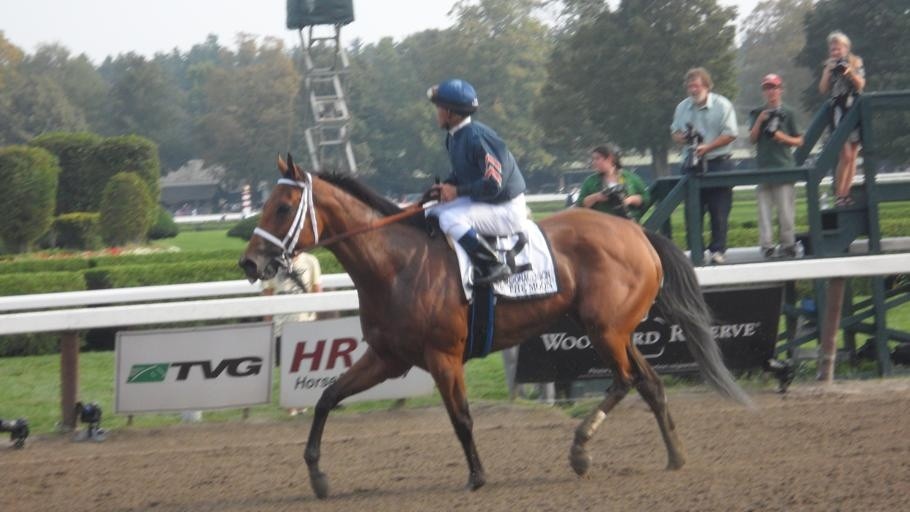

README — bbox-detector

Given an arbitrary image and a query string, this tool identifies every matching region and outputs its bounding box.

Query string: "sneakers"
[759,244,780,262]
[779,240,806,261]
[711,249,731,269]
[834,194,858,210]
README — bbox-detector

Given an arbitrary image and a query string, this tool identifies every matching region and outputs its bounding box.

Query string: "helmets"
[425,78,484,117]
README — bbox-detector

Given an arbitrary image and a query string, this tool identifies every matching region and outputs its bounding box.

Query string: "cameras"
[836,56,849,73]
[764,109,785,134]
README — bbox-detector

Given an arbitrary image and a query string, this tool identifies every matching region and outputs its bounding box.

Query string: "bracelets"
[843,67,851,75]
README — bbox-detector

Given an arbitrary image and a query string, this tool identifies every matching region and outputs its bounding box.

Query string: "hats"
[756,71,785,91]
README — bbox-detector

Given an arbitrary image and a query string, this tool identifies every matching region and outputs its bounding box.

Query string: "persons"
[670,67,738,265]
[260,251,324,417]
[575,144,652,222]
[818,32,866,210]
[746,73,804,258]
[426,78,526,287]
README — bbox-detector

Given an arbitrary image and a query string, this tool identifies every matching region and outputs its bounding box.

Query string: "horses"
[237,150,758,501]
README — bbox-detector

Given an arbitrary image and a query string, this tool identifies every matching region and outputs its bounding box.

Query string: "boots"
[456,225,513,290]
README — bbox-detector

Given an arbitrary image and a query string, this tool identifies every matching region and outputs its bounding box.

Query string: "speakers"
[286,0,354,29]
